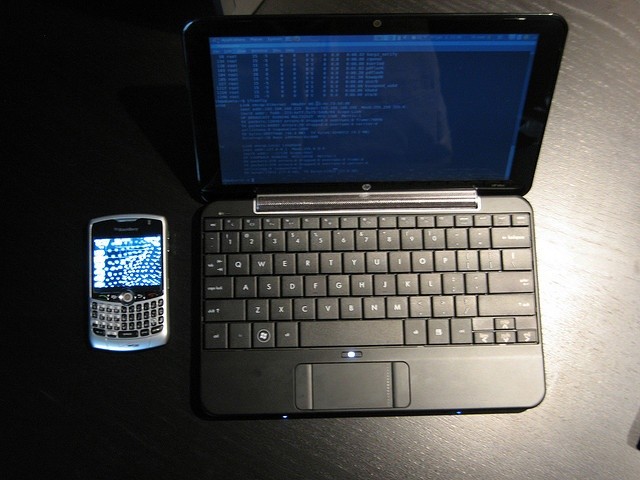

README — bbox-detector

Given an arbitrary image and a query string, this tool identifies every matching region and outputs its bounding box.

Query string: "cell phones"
[87,214,170,352]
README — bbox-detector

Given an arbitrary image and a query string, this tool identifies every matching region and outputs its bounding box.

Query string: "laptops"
[189,12,570,415]
